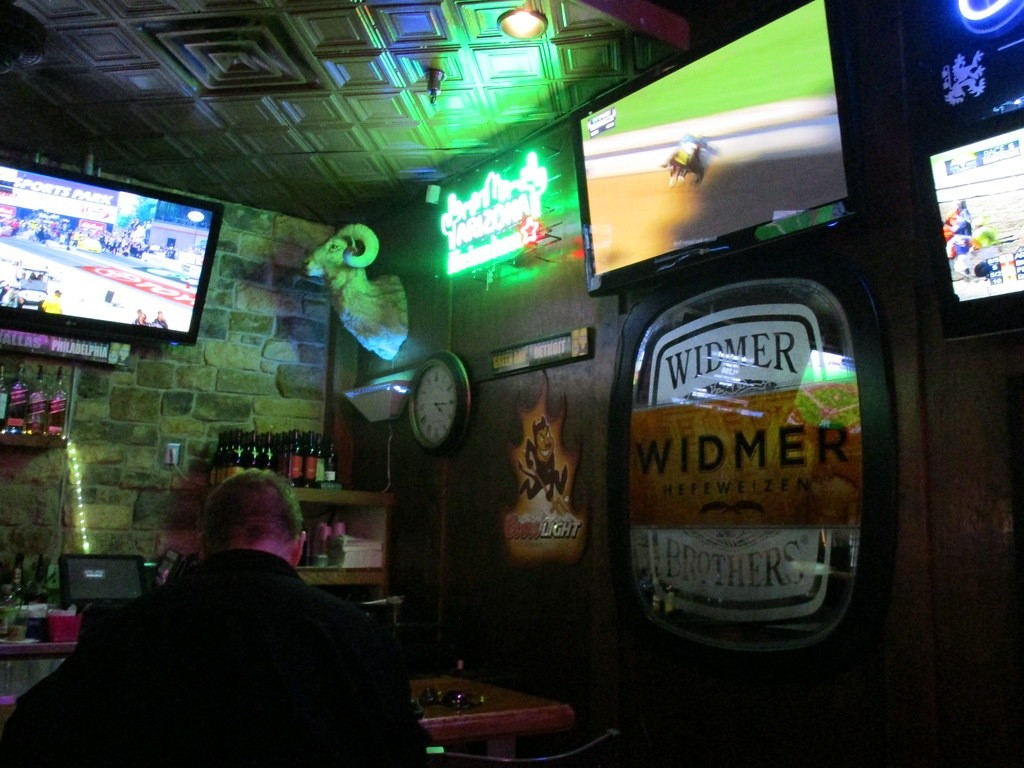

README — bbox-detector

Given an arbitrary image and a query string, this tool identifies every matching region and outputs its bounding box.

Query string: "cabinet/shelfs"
[0,642,77,729]
[214,487,391,584]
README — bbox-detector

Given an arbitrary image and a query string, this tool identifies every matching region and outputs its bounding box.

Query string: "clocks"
[407,350,470,447]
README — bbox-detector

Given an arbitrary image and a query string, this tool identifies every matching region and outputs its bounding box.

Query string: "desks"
[410,678,574,759]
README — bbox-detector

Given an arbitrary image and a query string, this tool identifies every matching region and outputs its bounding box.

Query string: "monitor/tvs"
[59,552,149,605]
[913,105,1024,339]
[564,1,865,297]
[0,153,227,345]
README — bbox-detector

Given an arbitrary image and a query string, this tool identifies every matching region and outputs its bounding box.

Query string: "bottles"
[0,365,10,433]
[6,362,28,434]
[209,429,336,489]
[25,365,46,435]
[0,552,50,643]
[46,368,66,437]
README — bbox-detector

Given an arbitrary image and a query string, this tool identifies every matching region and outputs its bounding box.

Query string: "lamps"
[498,9,548,41]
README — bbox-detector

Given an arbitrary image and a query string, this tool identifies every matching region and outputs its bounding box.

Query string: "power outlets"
[165,443,180,464]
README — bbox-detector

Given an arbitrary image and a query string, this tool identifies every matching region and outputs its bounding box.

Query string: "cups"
[48,615,81,642]
[299,520,344,567]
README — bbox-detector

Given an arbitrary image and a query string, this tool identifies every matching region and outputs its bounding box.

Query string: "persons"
[0,467,432,768]
[0,204,169,331]
